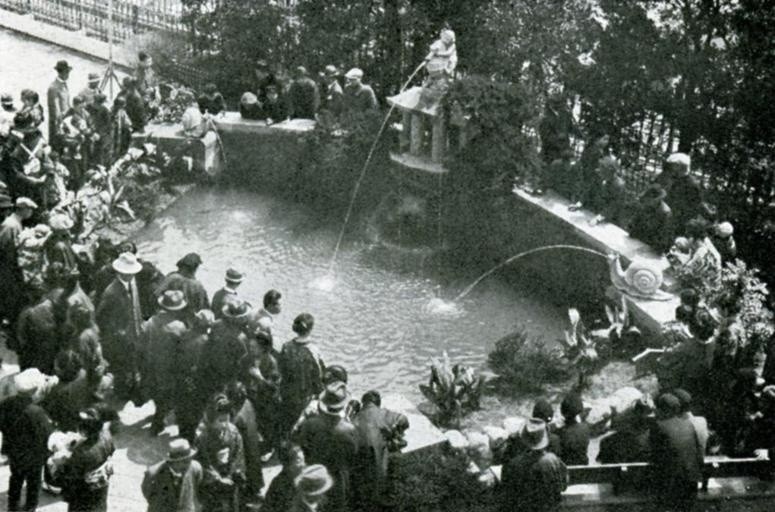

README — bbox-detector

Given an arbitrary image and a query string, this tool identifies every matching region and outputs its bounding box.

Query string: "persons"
[212,60,737,267]
[211,268,775,511]
[0,51,212,512]
[428,28,459,75]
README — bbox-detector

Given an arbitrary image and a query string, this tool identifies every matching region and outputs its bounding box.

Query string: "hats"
[163,438,198,461]
[519,418,549,450]
[631,395,657,419]
[113,251,143,274]
[158,253,252,326]
[346,69,364,82]
[294,464,333,496]
[16,197,40,209]
[48,214,74,229]
[319,381,351,408]
[641,184,666,203]
[1,61,103,143]
